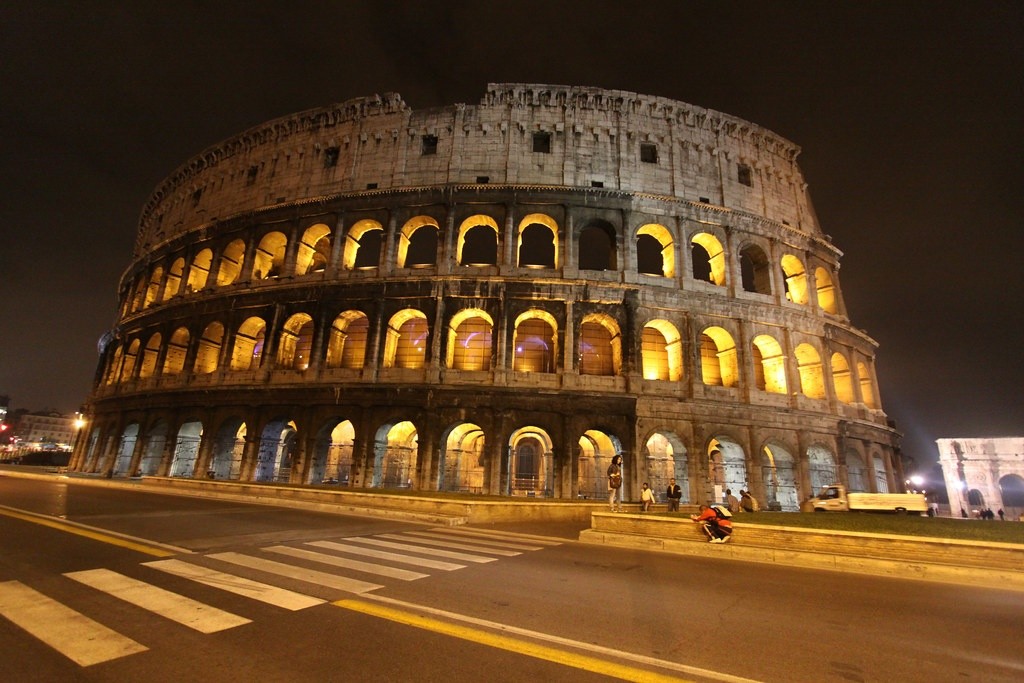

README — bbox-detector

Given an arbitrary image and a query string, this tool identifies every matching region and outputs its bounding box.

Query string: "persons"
[667,478,681,511]
[998,508,1004,520]
[727,489,739,513]
[690,505,734,543]
[746,491,758,512]
[738,490,752,513]
[928,507,994,520]
[641,483,655,512]
[607,455,627,513]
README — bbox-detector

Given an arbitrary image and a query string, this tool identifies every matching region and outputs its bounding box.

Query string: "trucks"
[804,484,928,514]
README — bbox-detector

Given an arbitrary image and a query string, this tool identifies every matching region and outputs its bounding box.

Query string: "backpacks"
[714,506,732,519]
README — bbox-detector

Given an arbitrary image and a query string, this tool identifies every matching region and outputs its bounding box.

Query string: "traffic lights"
[1,419,8,430]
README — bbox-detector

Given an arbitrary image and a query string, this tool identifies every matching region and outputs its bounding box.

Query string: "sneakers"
[618,508,627,512]
[709,538,721,543]
[609,509,617,513]
[721,536,730,543]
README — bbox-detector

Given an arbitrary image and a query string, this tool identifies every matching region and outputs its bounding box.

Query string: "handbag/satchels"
[610,476,622,488]
